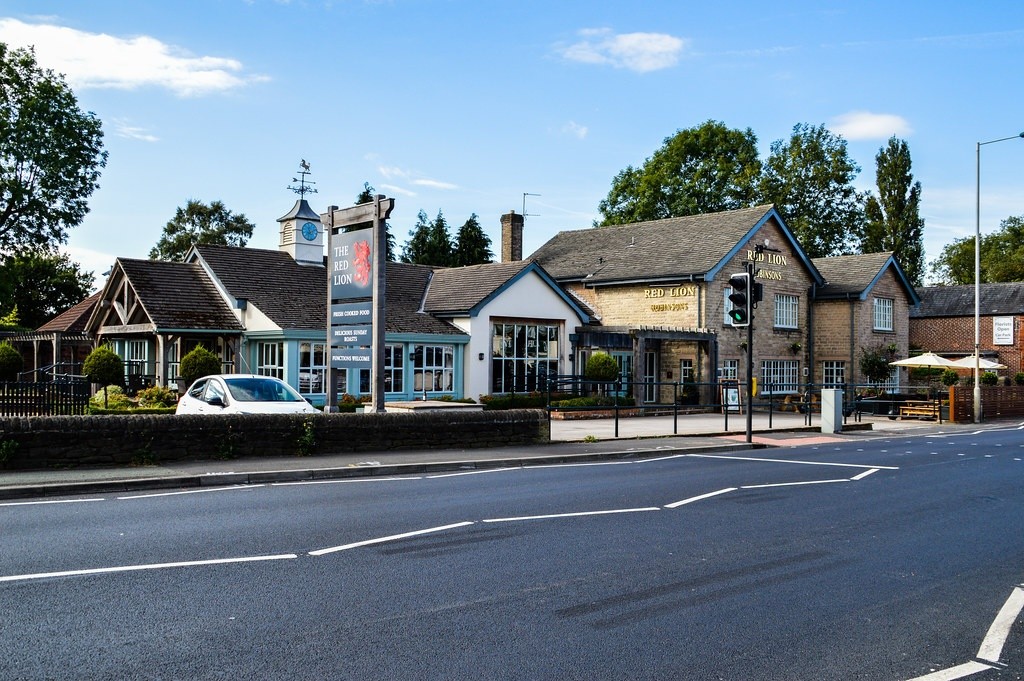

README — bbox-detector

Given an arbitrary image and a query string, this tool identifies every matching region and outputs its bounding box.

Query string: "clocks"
[302,223,317,241]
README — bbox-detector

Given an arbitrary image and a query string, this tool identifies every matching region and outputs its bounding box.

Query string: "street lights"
[973,131,1024,424]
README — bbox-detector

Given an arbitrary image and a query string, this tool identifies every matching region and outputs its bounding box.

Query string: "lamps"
[409,349,422,361]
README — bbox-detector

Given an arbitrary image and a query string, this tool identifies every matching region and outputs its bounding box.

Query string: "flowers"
[790,340,801,347]
[740,339,748,344]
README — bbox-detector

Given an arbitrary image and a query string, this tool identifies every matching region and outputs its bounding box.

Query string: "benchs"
[899,400,941,423]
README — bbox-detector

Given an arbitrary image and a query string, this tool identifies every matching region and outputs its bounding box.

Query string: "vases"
[738,344,748,353]
[791,347,801,356]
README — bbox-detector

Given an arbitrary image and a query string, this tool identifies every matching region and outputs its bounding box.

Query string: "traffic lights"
[728,272,750,327]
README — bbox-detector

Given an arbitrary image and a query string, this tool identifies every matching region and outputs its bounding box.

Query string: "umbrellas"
[953,353,1008,400]
[889,351,962,408]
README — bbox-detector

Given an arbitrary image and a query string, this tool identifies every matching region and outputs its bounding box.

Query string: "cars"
[175,373,325,415]
[300,372,319,389]
